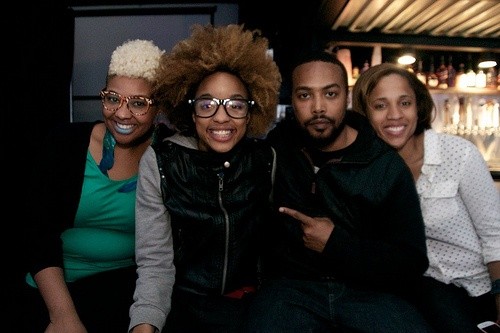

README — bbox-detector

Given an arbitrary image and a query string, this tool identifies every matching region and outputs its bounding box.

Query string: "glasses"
[189,97,254,119]
[100,88,155,116]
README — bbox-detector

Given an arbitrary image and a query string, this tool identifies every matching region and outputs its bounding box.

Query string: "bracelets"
[491,279,500,296]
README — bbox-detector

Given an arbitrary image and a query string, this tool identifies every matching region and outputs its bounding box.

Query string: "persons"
[0,38,182,333]
[260,51,429,333]
[127,23,286,333]
[351,63,500,333]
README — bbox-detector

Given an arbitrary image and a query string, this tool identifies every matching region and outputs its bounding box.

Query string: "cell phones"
[476,320,500,333]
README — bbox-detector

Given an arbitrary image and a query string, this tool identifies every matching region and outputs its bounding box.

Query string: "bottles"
[406,55,500,88]
[353,60,370,79]
